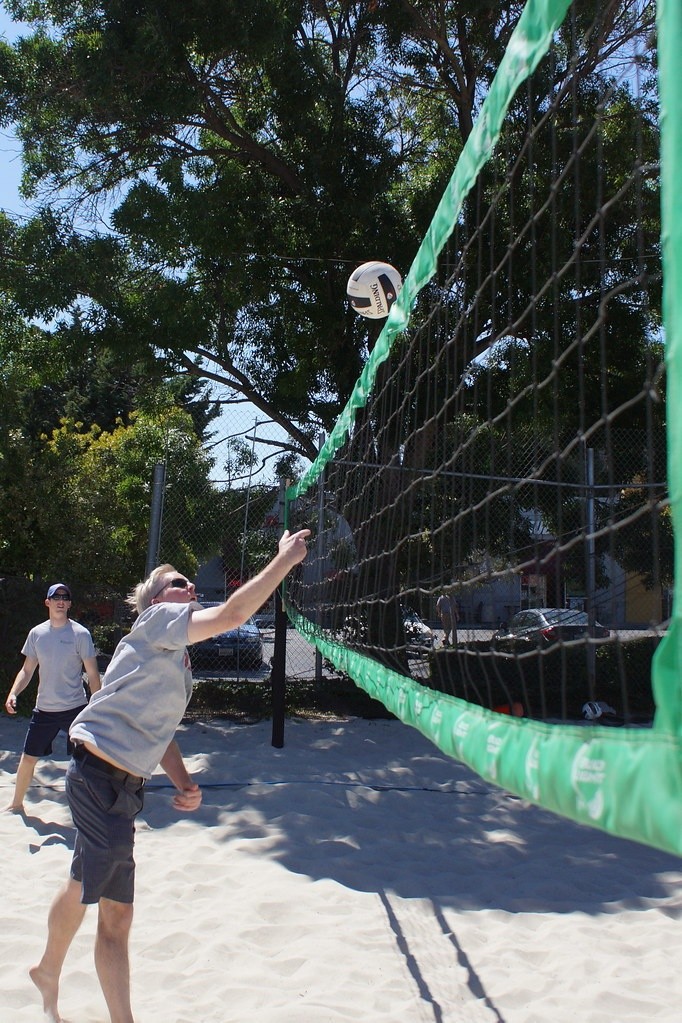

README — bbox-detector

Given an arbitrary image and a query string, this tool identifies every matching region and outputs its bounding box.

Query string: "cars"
[188,602,264,672]
[343,604,435,655]
[490,609,610,652]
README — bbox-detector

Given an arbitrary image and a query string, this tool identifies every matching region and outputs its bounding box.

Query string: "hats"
[47,583,71,601]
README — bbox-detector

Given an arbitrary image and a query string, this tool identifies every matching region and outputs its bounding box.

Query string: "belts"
[72,748,146,787]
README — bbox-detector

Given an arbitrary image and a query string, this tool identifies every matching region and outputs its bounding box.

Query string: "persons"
[5,584,100,814]
[29,529,313,1023]
[437,592,459,645]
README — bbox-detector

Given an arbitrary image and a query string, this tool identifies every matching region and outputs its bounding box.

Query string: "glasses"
[155,579,187,598]
[50,594,70,600]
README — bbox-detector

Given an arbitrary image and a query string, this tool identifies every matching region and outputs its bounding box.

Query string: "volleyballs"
[345,260,405,320]
